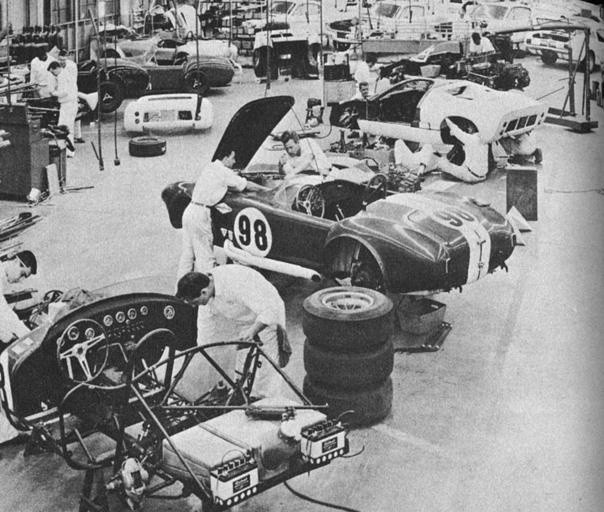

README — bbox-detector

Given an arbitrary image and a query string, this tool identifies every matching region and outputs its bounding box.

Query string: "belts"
[189,201,213,211]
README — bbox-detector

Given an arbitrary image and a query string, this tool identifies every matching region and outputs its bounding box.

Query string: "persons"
[0,249,38,447]
[347,82,376,138]
[432,117,490,183]
[29,47,78,157]
[173,144,267,274]
[176,263,286,402]
[470,31,496,54]
[278,131,330,175]
